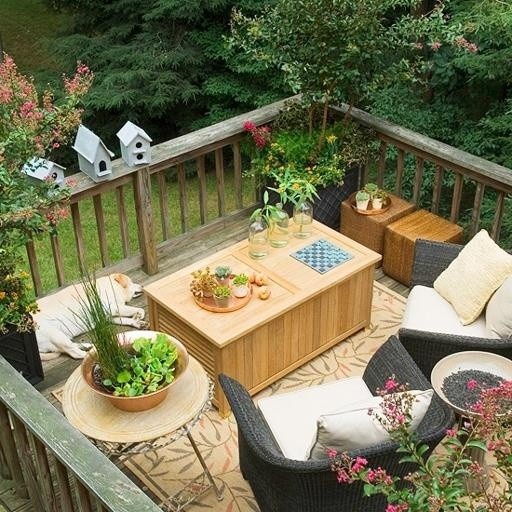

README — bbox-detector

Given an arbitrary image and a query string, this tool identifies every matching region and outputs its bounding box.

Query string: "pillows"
[305,388,434,460]
[433,229,512,325]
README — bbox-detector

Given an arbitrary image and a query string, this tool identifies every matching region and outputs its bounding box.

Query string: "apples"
[258,286,271,300]
[255,274,267,286]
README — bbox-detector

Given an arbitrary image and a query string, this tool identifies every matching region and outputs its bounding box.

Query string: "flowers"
[0,52,96,335]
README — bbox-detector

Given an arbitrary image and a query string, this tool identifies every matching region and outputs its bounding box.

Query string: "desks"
[62,354,224,512]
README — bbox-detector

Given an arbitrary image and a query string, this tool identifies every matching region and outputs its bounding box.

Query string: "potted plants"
[190,266,218,298]
[56,269,188,412]
[364,183,378,201]
[212,286,232,306]
[371,187,387,209]
[356,190,370,211]
[222,0,478,229]
[214,265,233,286]
[232,273,250,298]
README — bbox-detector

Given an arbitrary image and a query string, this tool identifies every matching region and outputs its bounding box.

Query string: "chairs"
[217,335,455,512]
[398,238,512,381]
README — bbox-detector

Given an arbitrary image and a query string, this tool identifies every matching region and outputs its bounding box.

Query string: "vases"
[0,325,44,386]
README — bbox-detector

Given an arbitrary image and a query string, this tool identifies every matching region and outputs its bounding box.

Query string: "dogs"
[25,273,150,360]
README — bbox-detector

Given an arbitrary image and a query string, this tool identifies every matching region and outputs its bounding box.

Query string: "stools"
[341,193,416,269]
[383,208,463,288]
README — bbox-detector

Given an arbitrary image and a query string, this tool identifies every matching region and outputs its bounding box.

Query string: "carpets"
[53,279,408,512]
